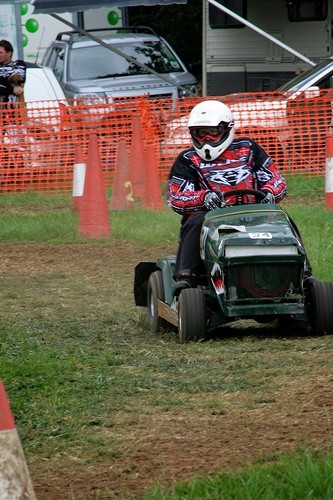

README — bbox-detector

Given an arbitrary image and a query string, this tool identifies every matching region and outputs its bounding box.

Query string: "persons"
[166,100,316,300]
[0,40,27,124]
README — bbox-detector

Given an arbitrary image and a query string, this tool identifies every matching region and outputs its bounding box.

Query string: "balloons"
[23,34,28,46]
[21,4,28,15]
[108,11,119,25]
[26,18,38,33]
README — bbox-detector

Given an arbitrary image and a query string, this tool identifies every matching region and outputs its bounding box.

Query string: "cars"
[159,54,333,175]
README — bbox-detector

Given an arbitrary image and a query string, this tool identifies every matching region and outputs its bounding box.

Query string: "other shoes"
[172,276,194,301]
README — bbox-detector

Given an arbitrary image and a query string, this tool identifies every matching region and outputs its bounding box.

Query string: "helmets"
[188,100,235,162]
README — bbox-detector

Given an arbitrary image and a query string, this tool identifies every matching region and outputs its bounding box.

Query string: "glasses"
[197,126,225,136]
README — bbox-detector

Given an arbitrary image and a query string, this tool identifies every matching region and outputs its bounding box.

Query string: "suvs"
[40,25,202,163]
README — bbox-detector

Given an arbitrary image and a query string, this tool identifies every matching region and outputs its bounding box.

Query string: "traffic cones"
[0,378,39,500]
[109,138,137,213]
[323,132,333,210]
[140,144,165,212]
[78,130,113,239]
[127,112,148,198]
[71,144,87,213]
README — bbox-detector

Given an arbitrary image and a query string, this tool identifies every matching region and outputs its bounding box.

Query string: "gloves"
[261,189,276,204]
[203,191,222,212]
